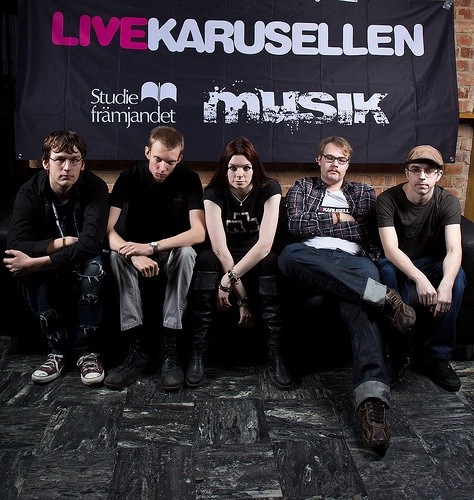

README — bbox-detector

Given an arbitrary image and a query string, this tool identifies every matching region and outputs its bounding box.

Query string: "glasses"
[48,156,83,165]
[406,167,440,175]
[320,153,349,165]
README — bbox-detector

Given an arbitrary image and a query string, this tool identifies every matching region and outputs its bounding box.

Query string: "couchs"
[0,196,474,358]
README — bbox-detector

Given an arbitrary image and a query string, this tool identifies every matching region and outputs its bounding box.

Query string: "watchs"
[151,242,158,254]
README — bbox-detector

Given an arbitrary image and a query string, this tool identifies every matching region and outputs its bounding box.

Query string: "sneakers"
[411,349,461,392]
[31,353,64,383]
[357,397,391,457]
[382,287,417,333]
[385,356,411,390]
[77,352,105,385]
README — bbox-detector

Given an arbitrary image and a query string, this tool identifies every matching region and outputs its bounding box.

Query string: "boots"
[257,276,294,388]
[185,270,221,387]
[103,324,150,389]
[157,325,185,390]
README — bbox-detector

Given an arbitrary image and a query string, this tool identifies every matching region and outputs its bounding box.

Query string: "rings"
[247,319,249,322]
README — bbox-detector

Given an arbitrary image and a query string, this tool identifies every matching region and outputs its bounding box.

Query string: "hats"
[407,144,445,172]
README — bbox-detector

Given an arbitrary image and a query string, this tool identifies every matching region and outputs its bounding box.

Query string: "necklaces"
[232,191,250,206]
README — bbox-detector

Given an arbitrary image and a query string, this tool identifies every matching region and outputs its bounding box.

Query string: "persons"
[279,136,416,451]
[184,137,293,389]
[104,127,206,391]
[3,130,112,385]
[377,146,467,391]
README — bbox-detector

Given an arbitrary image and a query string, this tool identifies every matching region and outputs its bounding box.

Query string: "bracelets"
[237,297,249,308]
[63,238,66,246]
[218,283,233,292]
[228,270,237,285]
[335,213,340,223]
